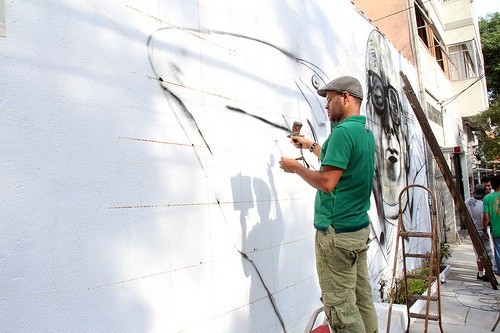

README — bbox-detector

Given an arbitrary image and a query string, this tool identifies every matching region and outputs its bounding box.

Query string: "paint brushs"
[281,114,300,136]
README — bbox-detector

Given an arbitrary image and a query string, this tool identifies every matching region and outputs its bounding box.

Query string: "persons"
[277,75,379,333]
[464,175,500,284]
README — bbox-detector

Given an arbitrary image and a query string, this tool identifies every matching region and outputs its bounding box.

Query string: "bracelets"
[309,143,318,152]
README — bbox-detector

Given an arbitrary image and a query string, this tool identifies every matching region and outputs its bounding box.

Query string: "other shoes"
[494,275,500,285]
[477,272,490,282]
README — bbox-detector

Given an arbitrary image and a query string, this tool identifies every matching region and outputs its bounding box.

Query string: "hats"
[472,184,486,197]
[317,75,363,100]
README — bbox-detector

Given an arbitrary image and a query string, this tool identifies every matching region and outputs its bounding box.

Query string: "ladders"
[398,70,500,291]
[386,184,445,333]
